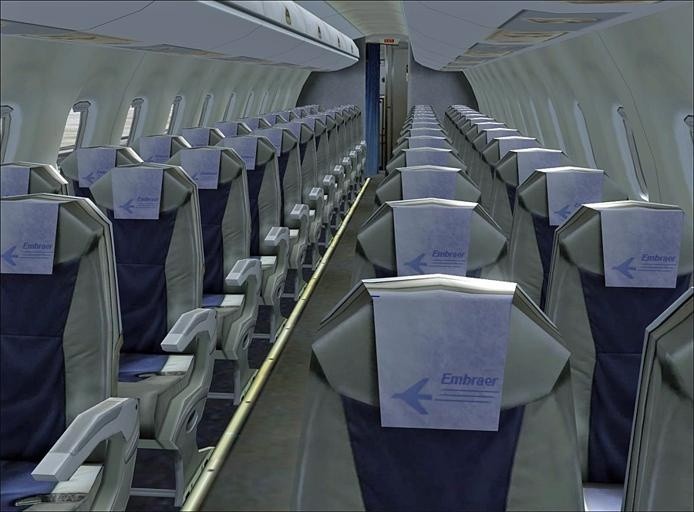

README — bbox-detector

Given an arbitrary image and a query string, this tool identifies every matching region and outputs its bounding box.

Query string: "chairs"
[340,106,365,187]
[620,302,694,510]
[133,135,190,166]
[293,118,343,231]
[210,121,250,136]
[513,164,631,313]
[392,135,458,154]
[275,111,299,122]
[333,108,362,195]
[324,110,357,204]
[547,199,692,484]
[300,105,316,115]
[214,136,291,343]
[470,129,524,184]
[0,162,73,197]
[86,163,219,509]
[351,200,514,286]
[399,103,496,122]
[262,114,288,125]
[373,165,484,204]
[290,275,585,510]
[270,121,325,268]
[401,122,447,129]
[348,104,367,185]
[455,118,497,147]
[293,108,308,118]
[384,147,467,171]
[462,121,508,163]
[490,148,573,246]
[310,114,351,216]
[59,146,144,200]
[243,117,271,128]
[481,136,546,212]
[178,126,222,145]
[1,194,142,512]
[398,127,448,139]
[161,146,267,389]
[246,127,310,299]
